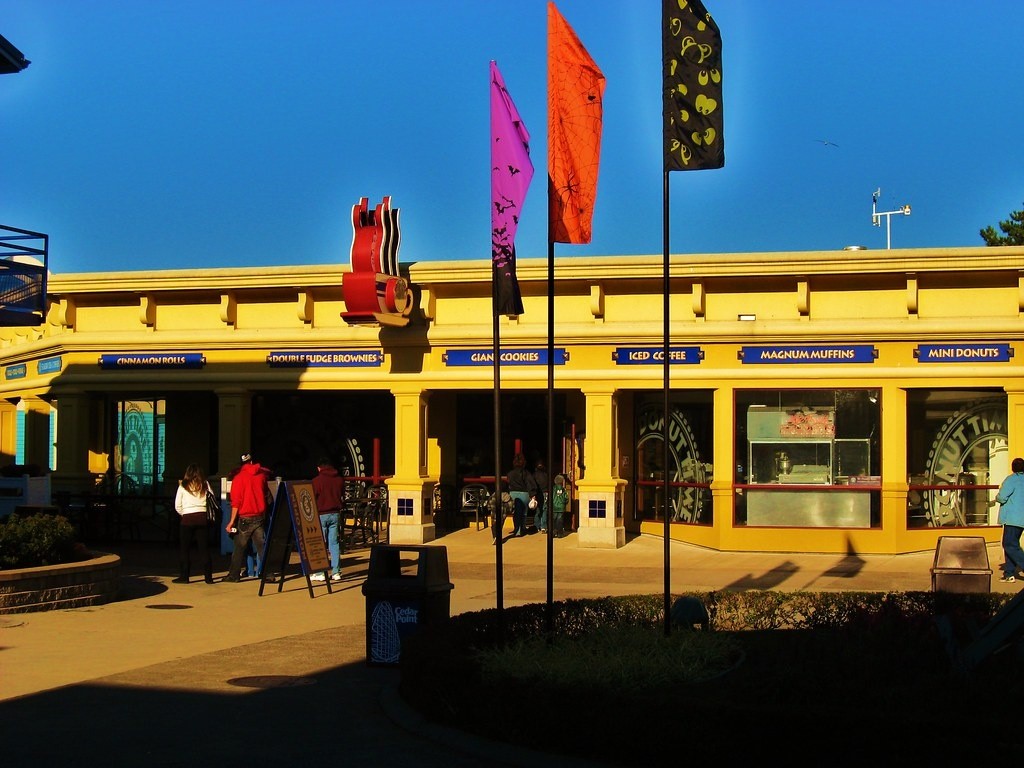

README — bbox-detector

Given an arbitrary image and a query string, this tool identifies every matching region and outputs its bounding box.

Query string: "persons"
[506,454,537,537]
[553,474,569,538]
[222,449,276,583]
[488,492,509,545]
[533,462,549,534]
[991,457,1024,583]
[310,457,346,582]
[172,462,215,584]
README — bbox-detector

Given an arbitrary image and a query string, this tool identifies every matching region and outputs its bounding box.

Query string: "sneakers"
[329,572,341,580]
[310,574,326,581]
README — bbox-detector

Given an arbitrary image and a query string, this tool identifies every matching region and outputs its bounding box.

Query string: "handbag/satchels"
[204,480,218,521]
[529,497,538,508]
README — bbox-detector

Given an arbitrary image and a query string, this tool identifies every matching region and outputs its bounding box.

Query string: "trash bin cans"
[362,542,455,666]
[929,535,994,594]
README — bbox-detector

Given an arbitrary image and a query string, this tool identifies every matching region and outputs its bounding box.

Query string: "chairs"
[449,483,488,533]
[342,482,365,512]
[362,483,389,531]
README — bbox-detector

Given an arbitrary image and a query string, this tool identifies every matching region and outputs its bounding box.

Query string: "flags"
[542,1,607,244]
[662,0,725,173]
[489,61,534,316]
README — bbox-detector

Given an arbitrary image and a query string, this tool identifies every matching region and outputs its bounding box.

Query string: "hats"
[241,451,253,464]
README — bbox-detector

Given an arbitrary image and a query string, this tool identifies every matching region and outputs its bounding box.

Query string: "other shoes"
[553,529,564,538]
[541,529,546,534]
[263,574,275,583]
[222,574,241,582]
[492,537,496,545]
[172,577,189,584]
[242,571,254,577]
[509,531,517,535]
[205,579,214,584]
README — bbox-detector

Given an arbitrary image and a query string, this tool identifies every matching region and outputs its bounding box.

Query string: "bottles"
[231,527,237,533]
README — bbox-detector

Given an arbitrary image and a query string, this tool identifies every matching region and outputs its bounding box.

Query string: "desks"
[341,498,386,549]
